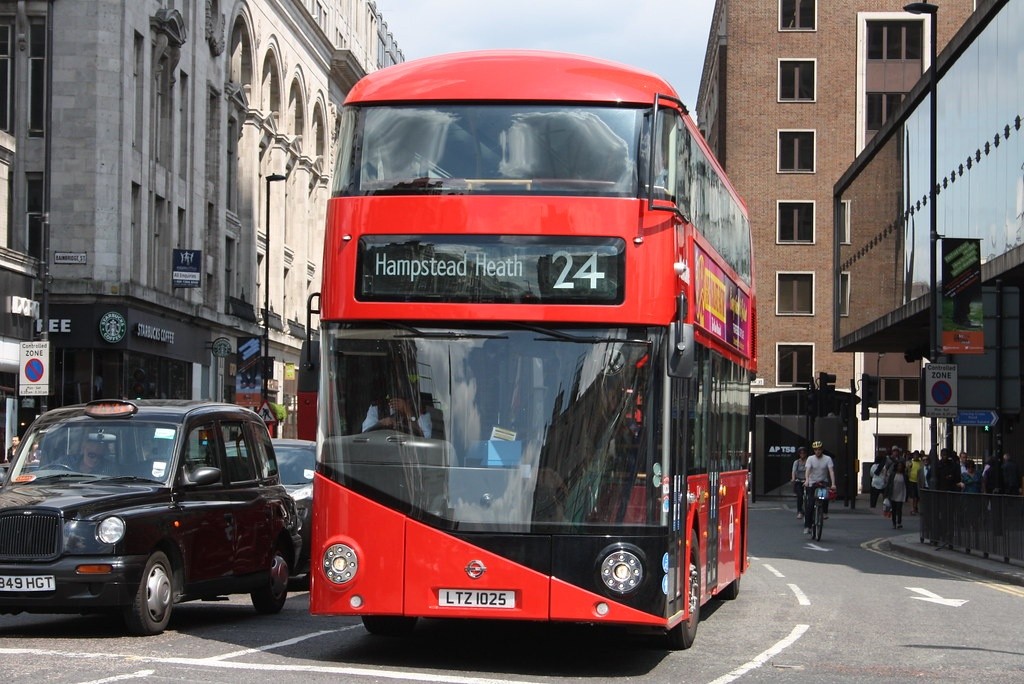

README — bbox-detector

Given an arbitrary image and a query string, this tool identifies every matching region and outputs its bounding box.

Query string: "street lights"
[261,170,288,430]
[874,351,886,462]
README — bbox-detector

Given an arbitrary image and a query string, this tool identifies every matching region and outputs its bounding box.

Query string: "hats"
[965,460,976,466]
[892,446,899,451]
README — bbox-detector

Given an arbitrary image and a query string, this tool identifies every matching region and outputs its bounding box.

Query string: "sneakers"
[823,513,829,519]
[797,511,803,518]
[804,527,810,534]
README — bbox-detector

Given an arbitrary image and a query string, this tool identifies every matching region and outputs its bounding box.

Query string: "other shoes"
[893,527,896,529]
[898,525,902,529]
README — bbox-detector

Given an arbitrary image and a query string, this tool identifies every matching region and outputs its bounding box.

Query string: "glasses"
[923,459,929,461]
[87,452,104,459]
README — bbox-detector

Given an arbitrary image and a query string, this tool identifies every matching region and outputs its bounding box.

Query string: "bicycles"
[803,480,836,543]
[790,477,808,523]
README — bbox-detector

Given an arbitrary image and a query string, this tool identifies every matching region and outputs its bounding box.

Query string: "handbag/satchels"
[883,498,892,512]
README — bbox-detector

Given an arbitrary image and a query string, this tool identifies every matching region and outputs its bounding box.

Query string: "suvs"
[0,397,304,636]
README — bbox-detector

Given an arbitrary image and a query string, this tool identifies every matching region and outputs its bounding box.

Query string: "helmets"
[812,441,822,448]
[798,447,807,453]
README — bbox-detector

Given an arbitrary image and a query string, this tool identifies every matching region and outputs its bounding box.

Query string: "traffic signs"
[950,410,999,427]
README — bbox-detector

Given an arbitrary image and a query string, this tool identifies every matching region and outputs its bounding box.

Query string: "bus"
[289,50,762,654]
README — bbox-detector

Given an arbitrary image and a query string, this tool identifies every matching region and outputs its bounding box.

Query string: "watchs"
[407,416,416,421]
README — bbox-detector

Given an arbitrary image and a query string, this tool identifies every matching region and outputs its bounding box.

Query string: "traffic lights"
[860,372,876,421]
[983,425,992,433]
[817,372,837,418]
[804,386,817,416]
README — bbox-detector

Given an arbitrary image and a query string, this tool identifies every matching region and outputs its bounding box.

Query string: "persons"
[793,438,1024,551]
[362,365,434,439]
[5,435,123,477]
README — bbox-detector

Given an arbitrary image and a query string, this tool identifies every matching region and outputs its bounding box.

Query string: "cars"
[200,438,317,574]
